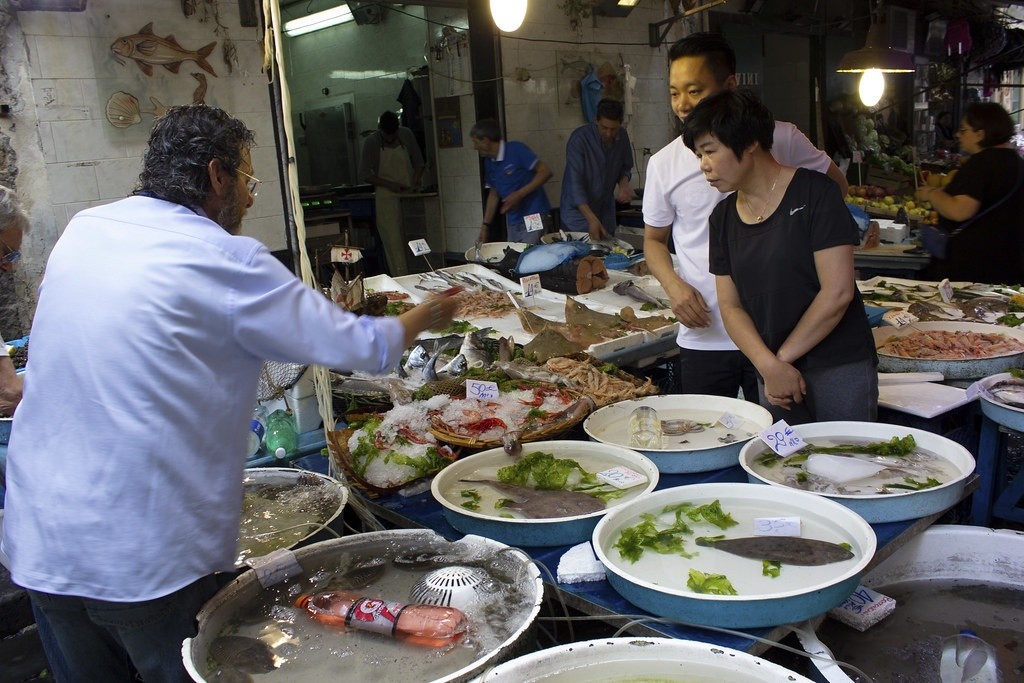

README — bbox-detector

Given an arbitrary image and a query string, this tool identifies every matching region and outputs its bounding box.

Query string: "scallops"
[205,550,523,683]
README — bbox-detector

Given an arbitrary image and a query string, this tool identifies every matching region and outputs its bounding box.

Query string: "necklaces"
[745,166,781,221]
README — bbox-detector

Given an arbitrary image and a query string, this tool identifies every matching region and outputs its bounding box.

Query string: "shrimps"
[887,328,1023,359]
[540,356,655,407]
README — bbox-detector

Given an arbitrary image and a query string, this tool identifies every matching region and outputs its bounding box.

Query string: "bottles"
[630,406,662,449]
[246,404,268,460]
[266,410,296,458]
[295,591,469,647]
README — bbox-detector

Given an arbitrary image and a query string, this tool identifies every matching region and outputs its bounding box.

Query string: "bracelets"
[423,299,441,328]
[483,223,489,226]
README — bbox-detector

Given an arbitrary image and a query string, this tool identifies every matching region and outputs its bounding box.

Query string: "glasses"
[234,166,265,199]
[0,236,21,265]
[959,128,983,136]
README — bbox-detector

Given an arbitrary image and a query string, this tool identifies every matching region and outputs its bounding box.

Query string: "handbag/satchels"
[921,223,947,257]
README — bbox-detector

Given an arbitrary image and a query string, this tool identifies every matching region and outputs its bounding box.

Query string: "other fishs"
[457,480,605,519]
[314,265,713,460]
[696,536,855,566]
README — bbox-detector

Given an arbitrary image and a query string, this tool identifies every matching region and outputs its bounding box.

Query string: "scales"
[300,191,336,211]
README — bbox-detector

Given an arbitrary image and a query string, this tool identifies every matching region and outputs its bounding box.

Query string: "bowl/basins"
[432,439,660,547]
[738,420,976,523]
[793,523,1024,683]
[871,320,1024,377]
[979,372,1024,433]
[584,393,773,474]
[461,636,812,683]
[180,518,545,682]
[214,464,347,570]
[590,481,876,628]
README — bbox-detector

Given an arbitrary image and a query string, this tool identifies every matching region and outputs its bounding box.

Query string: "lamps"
[597,0,642,18]
[281,2,355,38]
[834,0,917,107]
[489,0,527,33]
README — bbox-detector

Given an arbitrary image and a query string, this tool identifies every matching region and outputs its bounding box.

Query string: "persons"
[560,98,633,244]
[359,110,427,276]
[913,102,1024,287]
[471,118,554,242]
[935,112,957,152]
[641,32,849,402]
[0,185,28,418]
[681,89,879,424]
[0,104,460,683]
